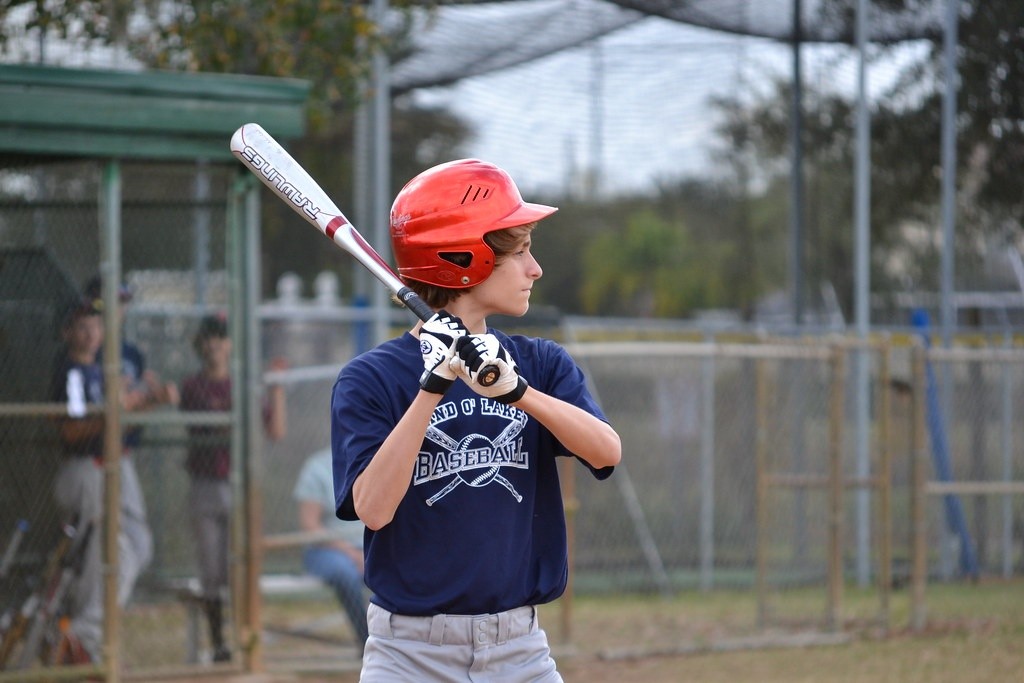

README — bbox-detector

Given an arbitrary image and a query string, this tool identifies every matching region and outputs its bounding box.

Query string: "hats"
[68,295,103,312]
[86,275,131,302]
[201,314,227,336]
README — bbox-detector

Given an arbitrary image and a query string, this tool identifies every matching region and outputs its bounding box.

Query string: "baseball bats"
[229,121,501,388]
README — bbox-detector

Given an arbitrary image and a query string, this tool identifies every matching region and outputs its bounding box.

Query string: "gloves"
[450,332,527,406]
[418,309,470,396]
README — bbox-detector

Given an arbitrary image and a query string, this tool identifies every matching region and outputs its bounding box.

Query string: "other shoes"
[214,648,229,662]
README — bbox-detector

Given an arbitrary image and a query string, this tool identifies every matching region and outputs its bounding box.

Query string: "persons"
[329,158,623,683]
[178,309,288,664]
[293,441,369,659]
[46,270,181,664]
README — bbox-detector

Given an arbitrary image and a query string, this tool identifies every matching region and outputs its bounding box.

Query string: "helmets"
[389,158,558,288]
